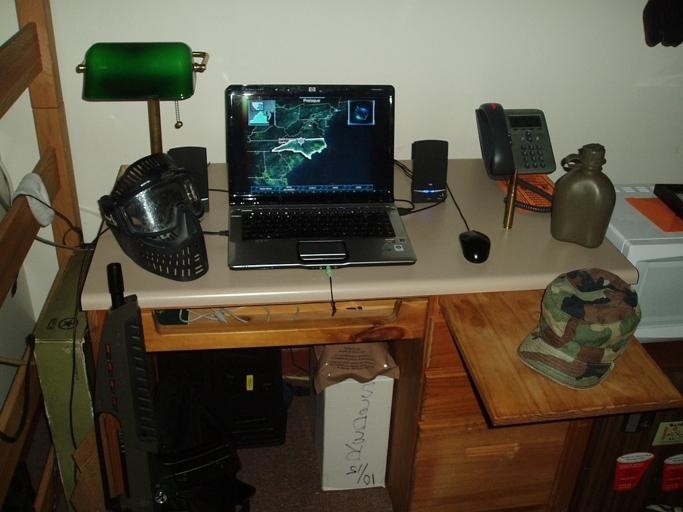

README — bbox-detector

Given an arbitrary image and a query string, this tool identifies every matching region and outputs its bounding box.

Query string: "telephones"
[476,103,556,180]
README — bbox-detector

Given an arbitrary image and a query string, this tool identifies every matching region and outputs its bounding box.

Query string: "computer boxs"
[156,346,286,449]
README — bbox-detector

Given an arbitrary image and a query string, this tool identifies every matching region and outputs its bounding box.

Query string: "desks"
[80,159,681,511]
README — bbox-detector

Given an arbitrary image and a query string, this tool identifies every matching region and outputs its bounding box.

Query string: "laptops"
[225,85,417,271]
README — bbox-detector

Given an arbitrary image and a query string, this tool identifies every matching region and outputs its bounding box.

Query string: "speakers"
[168,147,209,212]
[411,140,448,203]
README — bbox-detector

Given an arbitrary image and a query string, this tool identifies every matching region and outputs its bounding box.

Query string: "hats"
[516,268,641,391]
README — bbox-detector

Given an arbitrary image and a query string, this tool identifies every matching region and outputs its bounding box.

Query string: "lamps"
[75,41,210,155]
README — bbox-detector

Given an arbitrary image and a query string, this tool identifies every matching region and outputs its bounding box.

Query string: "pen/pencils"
[503,168,518,230]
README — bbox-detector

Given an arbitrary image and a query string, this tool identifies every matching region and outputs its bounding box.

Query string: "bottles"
[549,140,618,247]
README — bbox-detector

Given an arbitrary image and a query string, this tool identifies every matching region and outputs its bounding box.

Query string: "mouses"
[459,230,490,263]
